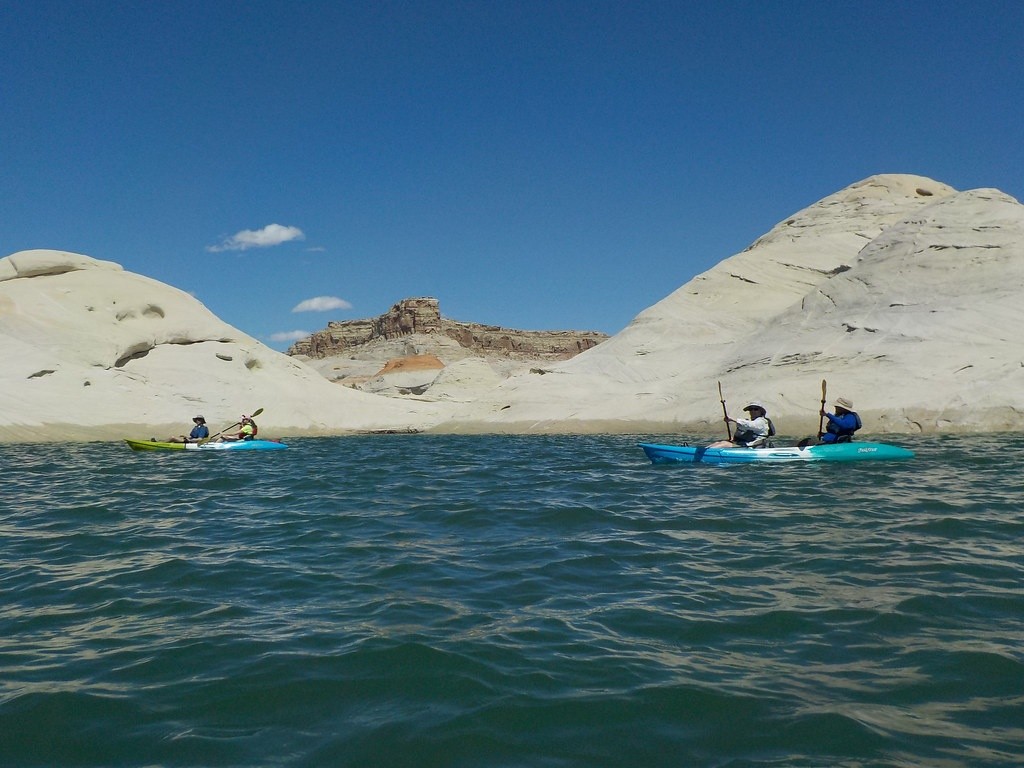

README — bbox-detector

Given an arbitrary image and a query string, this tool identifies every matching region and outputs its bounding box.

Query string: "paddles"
[819,379,827,435]
[718,380,732,442]
[197,407,264,447]
[179,435,186,439]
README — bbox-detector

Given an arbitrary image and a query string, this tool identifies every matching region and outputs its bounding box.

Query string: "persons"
[684,401,770,448]
[215,413,255,443]
[797,395,863,446]
[151,415,210,443]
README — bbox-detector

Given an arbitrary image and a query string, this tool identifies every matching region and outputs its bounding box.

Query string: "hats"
[192,415,206,423]
[743,401,767,412]
[831,397,856,412]
[239,414,251,420]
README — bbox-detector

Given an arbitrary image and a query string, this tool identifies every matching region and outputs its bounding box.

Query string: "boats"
[635,439,917,464]
[122,438,291,452]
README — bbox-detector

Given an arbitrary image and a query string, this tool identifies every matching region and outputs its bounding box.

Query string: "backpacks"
[836,412,862,436]
[204,426,209,437]
[243,424,257,435]
[752,416,775,438]
